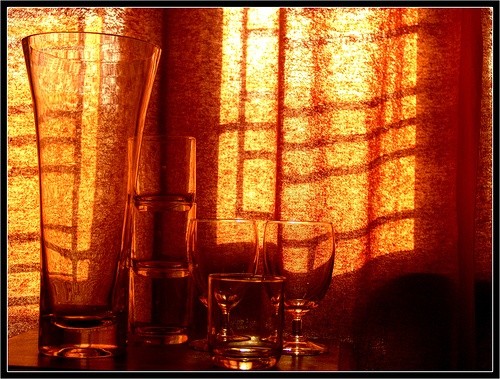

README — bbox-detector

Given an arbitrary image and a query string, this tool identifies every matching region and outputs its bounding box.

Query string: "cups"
[131,259,192,346]
[126,136,196,212]
[127,199,197,279]
[208,272,286,372]
[22,31,162,358]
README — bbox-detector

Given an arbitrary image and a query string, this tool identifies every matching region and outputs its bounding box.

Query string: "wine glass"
[262,219,337,355]
[186,219,259,343]
